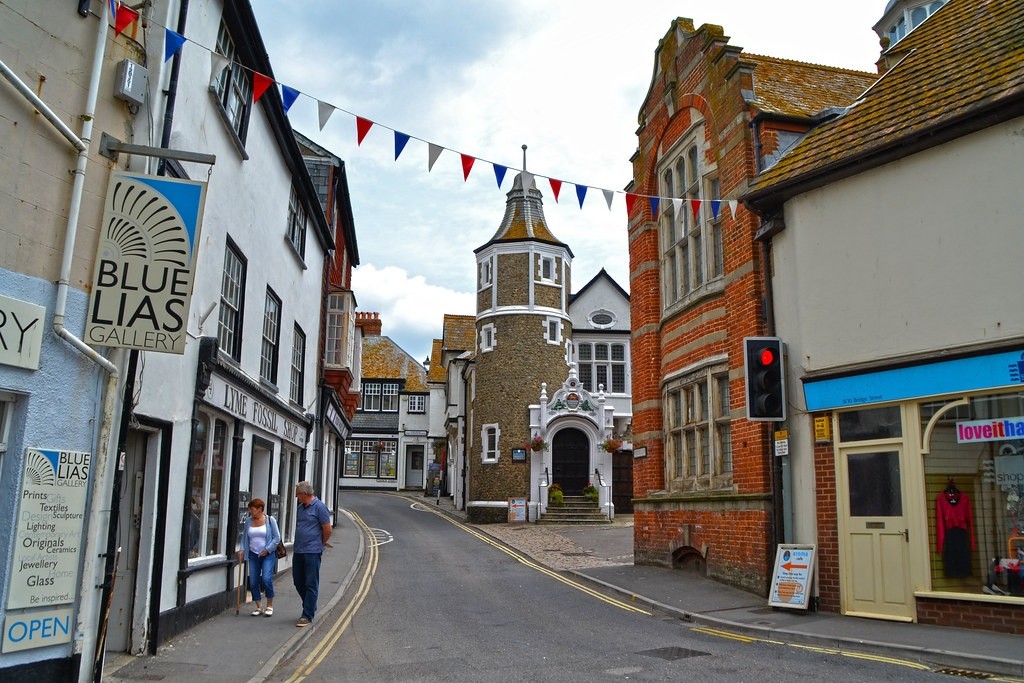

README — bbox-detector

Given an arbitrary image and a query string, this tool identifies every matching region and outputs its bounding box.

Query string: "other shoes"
[250,607,264,616]
[265,606,274,616]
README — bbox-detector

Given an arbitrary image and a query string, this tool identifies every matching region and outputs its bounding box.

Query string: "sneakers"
[296,617,312,626]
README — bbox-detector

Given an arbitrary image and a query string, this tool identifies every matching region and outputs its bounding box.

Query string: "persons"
[293,479,333,626]
[237,498,282,616]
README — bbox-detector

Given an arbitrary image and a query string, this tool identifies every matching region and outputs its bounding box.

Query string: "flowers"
[599,439,623,452]
[529,436,546,447]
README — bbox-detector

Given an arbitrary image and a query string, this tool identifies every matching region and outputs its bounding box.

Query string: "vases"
[607,448,616,453]
[533,445,542,451]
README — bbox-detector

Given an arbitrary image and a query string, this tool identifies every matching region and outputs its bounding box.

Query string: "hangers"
[944,481,961,494]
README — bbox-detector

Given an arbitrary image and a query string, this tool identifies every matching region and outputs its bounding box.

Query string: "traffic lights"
[744,336,787,422]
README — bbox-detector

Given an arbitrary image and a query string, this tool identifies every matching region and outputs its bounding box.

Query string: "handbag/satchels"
[268,515,287,559]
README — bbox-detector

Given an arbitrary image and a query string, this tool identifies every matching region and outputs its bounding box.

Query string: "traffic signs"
[768,543,820,619]
